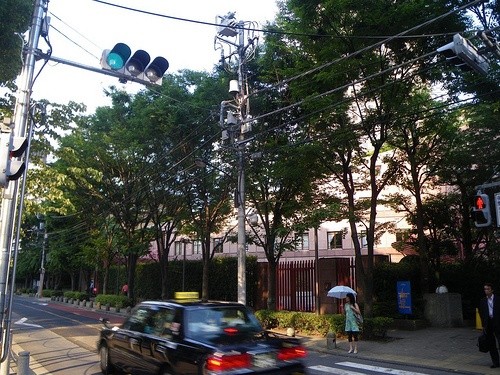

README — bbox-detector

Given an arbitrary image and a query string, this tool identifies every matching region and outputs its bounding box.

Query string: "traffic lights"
[101,42,169,85]
[472,194,492,227]
[0,132,29,187]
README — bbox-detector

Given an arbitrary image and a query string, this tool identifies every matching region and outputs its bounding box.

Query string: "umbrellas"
[327,286,357,305]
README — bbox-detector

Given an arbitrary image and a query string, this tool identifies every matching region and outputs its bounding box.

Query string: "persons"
[340,293,360,353]
[89,281,94,298]
[478,283,500,367]
[122,282,128,296]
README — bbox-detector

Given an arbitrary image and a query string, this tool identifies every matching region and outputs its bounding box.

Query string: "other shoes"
[354,351,358,354]
[347,348,354,353]
[490,364,500,368]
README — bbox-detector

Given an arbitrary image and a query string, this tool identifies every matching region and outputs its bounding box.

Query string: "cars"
[96,291,308,375]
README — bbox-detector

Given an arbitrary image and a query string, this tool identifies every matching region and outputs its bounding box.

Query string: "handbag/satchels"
[356,314,363,324]
[478,333,489,353]
